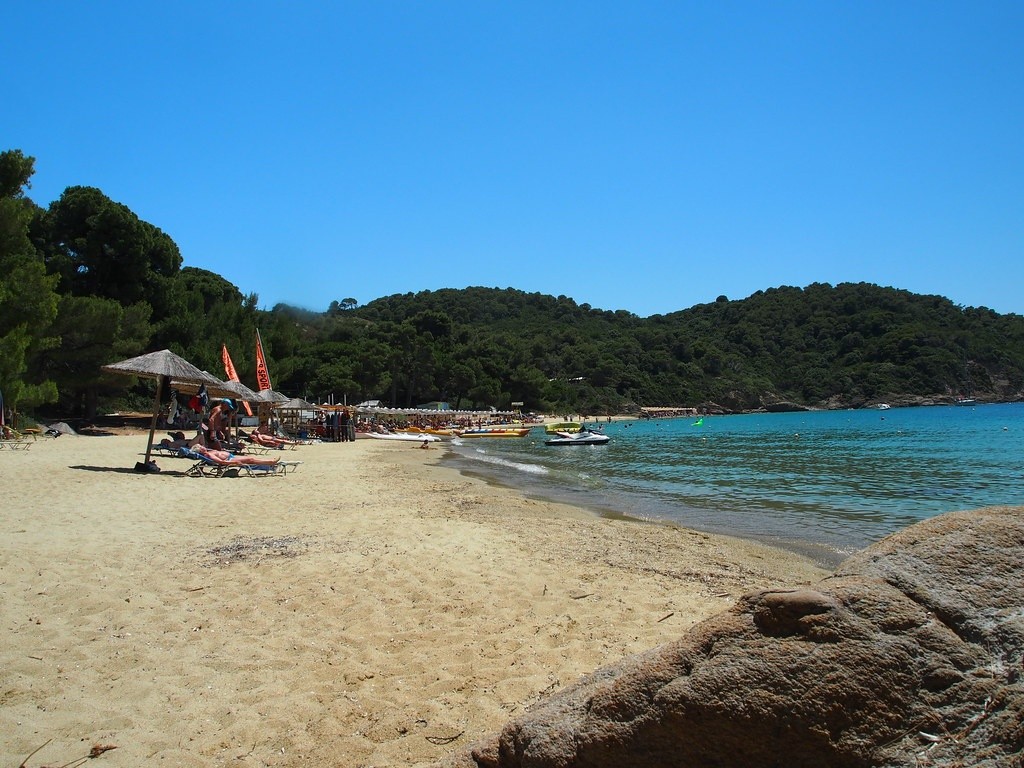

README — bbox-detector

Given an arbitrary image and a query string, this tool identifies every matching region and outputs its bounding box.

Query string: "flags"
[189,381,208,411]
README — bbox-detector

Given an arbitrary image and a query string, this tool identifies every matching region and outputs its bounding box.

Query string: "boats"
[364,430,442,442]
[692,418,704,427]
[451,428,531,437]
[543,429,611,446]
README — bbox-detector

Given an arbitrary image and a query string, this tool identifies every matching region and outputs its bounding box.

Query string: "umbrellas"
[96,349,519,462]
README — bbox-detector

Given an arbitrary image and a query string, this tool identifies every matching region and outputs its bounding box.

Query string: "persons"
[159,397,708,467]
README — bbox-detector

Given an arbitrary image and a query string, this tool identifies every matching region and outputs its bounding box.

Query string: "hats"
[222,398,233,409]
